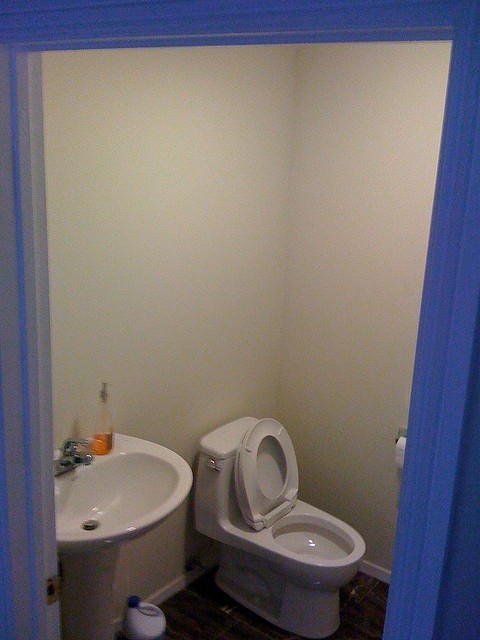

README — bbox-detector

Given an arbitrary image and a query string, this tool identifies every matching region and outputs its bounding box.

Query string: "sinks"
[53,430,194,552]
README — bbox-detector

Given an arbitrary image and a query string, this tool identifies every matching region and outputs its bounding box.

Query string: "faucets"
[54,436,92,476]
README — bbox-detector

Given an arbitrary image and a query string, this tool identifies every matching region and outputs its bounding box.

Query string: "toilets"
[193,416,367,640]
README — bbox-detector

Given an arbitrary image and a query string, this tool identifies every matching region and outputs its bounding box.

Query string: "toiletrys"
[92,382,113,456]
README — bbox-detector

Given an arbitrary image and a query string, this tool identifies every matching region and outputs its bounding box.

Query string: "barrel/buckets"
[124,595,166,639]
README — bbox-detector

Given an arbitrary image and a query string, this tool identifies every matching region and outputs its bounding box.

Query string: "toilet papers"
[394,436,407,468]
